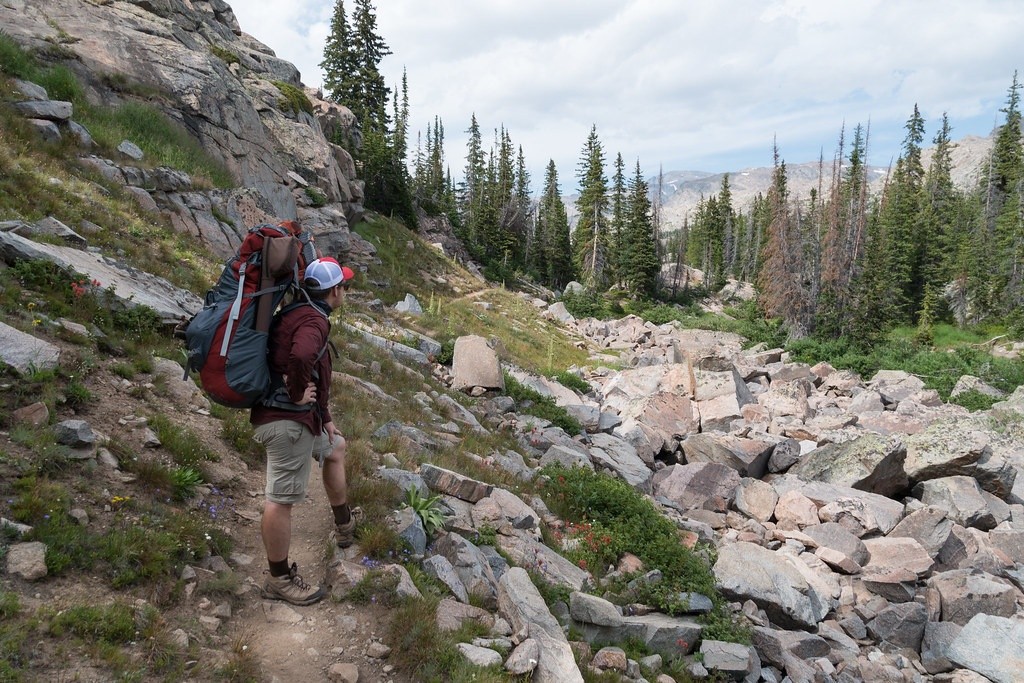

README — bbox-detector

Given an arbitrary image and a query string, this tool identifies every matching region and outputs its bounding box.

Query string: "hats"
[303,257,355,290]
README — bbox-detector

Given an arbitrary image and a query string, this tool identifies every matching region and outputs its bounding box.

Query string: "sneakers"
[262,562,322,606]
[333,506,366,547]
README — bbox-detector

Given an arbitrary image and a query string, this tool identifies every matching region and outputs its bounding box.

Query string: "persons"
[250,257,366,603]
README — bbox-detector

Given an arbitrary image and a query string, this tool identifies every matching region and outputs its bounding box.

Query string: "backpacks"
[187,218,332,411]
